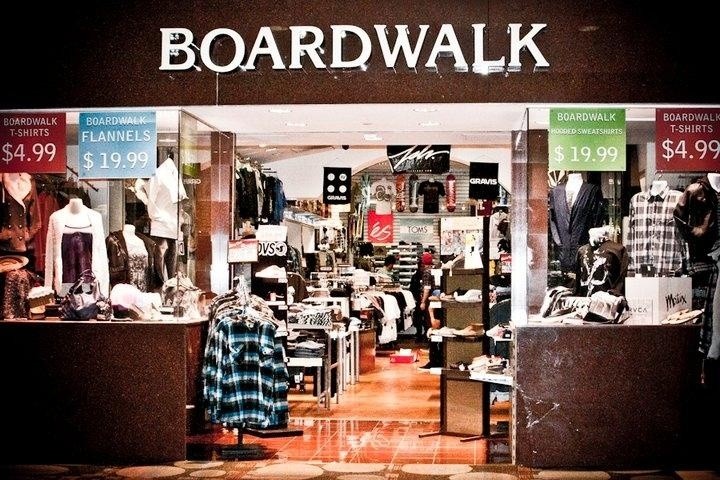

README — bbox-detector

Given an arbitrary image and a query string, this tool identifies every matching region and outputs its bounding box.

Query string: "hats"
[420,251,432,265]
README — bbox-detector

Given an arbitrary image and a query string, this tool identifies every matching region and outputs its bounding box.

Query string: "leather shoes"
[453,326,477,336]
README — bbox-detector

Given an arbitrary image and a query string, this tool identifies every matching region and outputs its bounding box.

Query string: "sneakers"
[450,361,469,370]
[418,361,441,371]
[455,289,483,304]
[539,284,571,317]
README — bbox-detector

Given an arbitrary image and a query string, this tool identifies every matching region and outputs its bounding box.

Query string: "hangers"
[214,277,276,332]
[61,170,100,198]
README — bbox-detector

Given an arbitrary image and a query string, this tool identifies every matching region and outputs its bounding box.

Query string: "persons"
[0,173,41,253]
[549,174,601,273]
[674,173,720,272]
[419,253,438,372]
[629,181,683,277]
[43,197,109,300]
[408,261,427,343]
[377,254,396,281]
[106,226,156,293]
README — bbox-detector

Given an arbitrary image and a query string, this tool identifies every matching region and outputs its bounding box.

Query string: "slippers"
[660,308,702,325]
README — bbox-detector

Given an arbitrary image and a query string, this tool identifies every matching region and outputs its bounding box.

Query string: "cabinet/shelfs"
[439,208,509,435]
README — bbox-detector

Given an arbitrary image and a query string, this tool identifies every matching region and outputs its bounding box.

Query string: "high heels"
[440,252,465,271]
